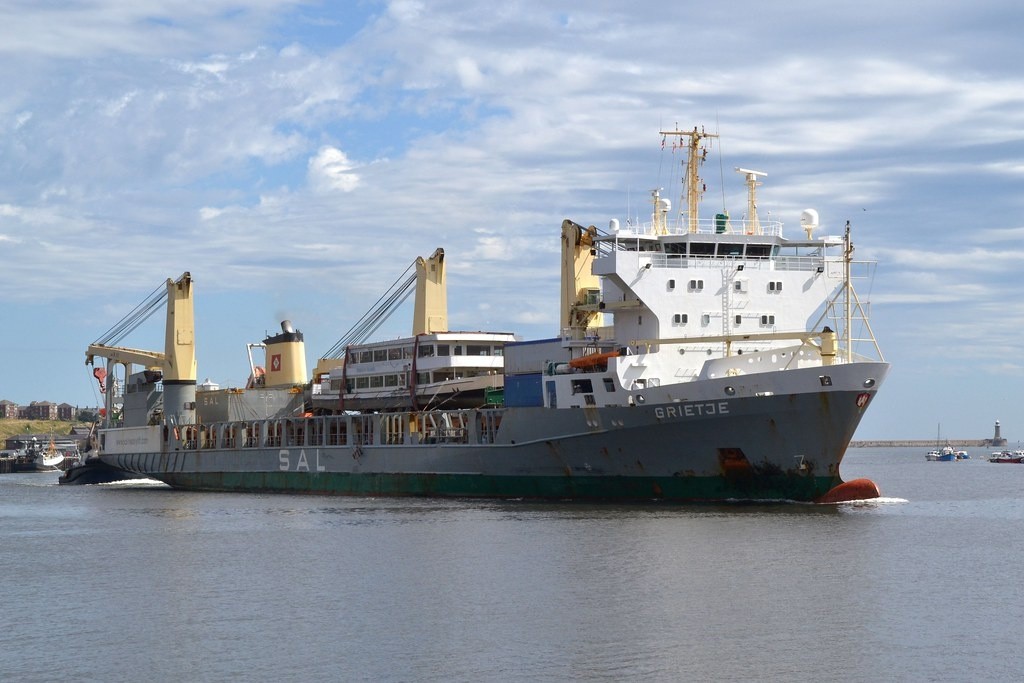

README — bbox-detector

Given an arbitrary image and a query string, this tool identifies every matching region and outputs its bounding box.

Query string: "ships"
[83,124,891,505]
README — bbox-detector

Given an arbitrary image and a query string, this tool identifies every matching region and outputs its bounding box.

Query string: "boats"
[925,446,970,461]
[989,440,1024,463]
[35,424,65,472]
[568,349,621,370]
